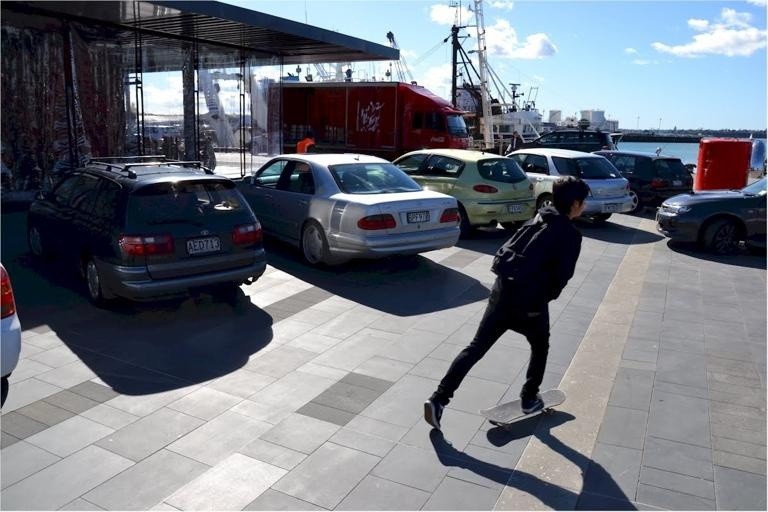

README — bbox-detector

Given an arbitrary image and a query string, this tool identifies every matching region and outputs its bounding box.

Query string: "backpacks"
[489,214,565,283]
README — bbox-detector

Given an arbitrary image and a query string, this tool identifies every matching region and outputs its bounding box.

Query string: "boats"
[385,24,623,154]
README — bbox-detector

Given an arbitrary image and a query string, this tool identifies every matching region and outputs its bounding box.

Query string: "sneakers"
[521,394,544,413]
[424,399,442,431]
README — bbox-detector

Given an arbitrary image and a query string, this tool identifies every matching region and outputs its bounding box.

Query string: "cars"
[1,257,23,379]
[654,173,767,256]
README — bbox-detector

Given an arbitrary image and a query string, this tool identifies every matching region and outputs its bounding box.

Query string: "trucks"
[265,81,469,162]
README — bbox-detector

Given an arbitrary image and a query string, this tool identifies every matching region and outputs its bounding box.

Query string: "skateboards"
[479,390,566,431]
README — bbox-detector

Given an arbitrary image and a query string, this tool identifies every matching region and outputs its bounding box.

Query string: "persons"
[423,175,591,431]
[295,130,316,153]
[510,130,523,151]
[762,158,766,177]
